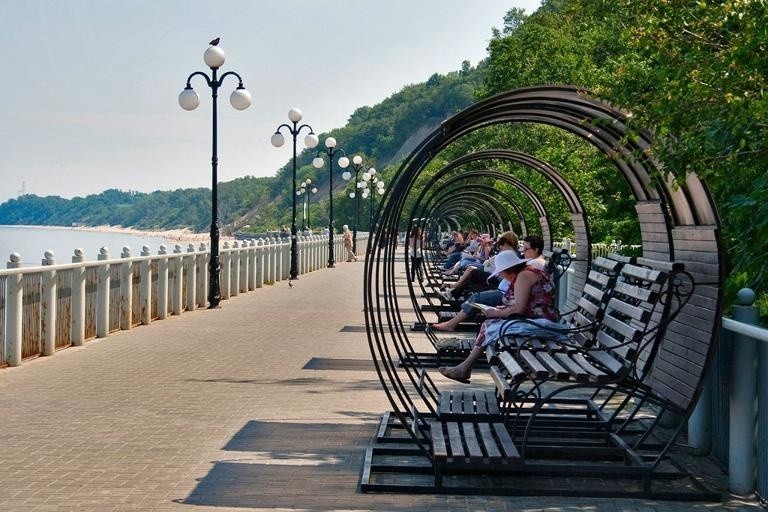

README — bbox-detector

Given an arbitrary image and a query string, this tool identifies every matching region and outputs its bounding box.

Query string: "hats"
[476,234,493,243]
[486,250,528,280]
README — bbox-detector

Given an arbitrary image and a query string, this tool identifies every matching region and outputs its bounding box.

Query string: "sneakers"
[433,287,451,303]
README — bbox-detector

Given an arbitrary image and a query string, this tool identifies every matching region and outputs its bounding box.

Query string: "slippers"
[438,366,471,384]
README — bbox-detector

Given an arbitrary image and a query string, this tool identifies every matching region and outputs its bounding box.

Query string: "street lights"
[343,155,385,255]
[271,110,319,280]
[179,37,251,308]
[313,136,349,268]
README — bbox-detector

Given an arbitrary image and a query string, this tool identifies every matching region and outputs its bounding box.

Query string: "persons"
[343,225,358,262]
[433,229,558,384]
[280,225,330,238]
[408,226,424,282]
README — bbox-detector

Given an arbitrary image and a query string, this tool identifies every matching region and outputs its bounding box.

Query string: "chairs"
[427,250,684,489]
